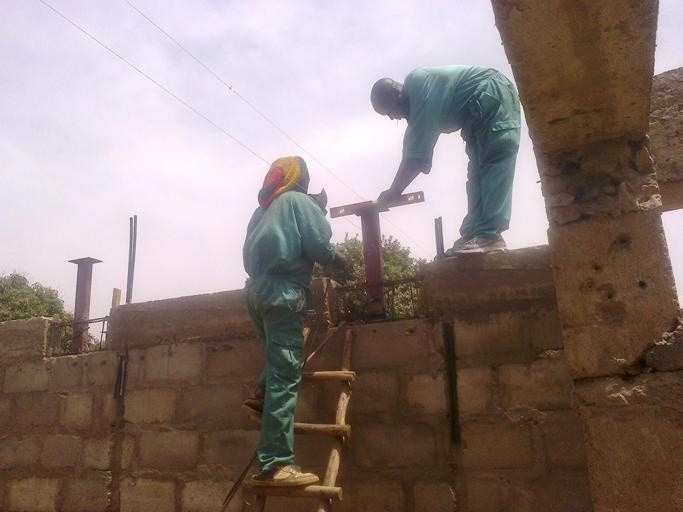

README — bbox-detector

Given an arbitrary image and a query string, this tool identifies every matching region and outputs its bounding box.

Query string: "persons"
[370,64,523,259]
[240,154,355,489]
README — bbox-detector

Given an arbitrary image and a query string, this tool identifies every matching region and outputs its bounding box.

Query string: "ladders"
[240,327,358,512]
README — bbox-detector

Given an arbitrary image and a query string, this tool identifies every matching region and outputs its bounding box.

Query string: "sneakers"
[249,464,320,487]
[445,234,506,257]
[243,396,263,423]
